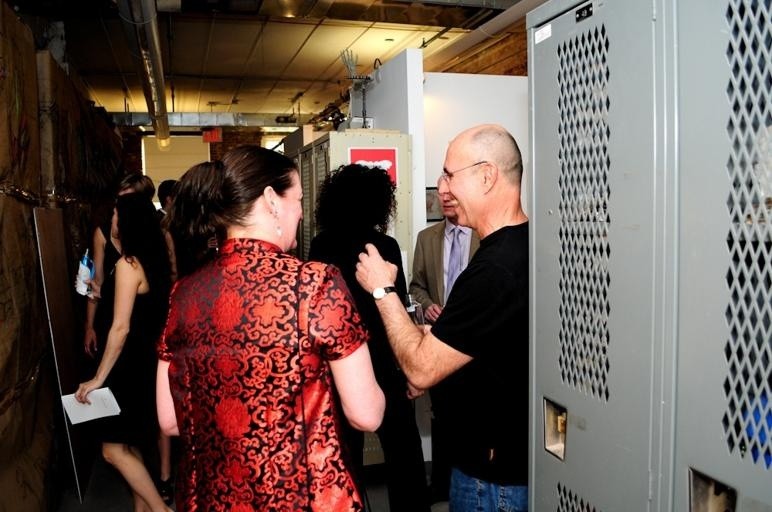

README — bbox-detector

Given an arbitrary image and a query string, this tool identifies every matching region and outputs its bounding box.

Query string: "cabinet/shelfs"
[287,149,303,263]
[314,129,411,466]
[303,140,315,260]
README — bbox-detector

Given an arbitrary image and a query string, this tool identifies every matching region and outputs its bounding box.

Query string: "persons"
[352,122,531,511]
[69,162,481,511]
[156,142,389,511]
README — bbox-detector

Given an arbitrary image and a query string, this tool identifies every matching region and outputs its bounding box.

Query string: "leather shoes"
[157,478,174,507]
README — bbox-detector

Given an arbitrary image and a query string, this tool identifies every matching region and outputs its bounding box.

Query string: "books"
[58,387,125,426]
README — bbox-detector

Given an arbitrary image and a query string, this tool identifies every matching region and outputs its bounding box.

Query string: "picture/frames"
[426,186,446,222]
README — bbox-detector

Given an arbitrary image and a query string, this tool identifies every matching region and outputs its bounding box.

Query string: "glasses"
[441,161,488,184]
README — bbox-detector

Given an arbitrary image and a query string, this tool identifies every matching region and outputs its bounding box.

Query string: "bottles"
[404,294,424,332]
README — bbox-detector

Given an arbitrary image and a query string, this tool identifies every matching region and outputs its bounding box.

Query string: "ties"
[444,228,464,305]
